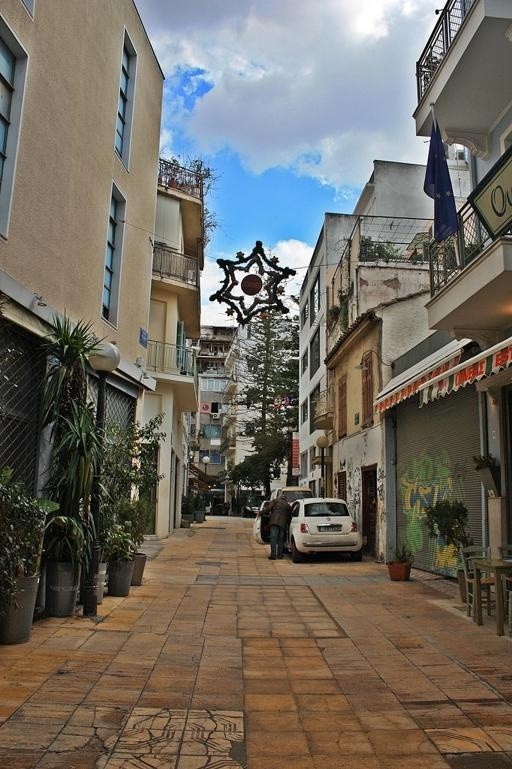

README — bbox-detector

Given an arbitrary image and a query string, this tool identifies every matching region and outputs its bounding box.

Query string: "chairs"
[498,546,511,622]
[460,546,505,620]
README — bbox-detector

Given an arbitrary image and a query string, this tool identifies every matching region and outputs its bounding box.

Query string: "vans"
[277,486,314,512]
[270,488,282,501]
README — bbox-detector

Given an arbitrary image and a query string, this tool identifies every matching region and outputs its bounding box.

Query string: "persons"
[257,495,291,559]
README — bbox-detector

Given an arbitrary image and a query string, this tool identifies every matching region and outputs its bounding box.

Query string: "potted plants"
[388,540,414,582]
[1,308,166,644]
[424,500,480,602]
[183,491,208,523]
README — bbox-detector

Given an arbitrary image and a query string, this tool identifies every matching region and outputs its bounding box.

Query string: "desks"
[472,559,512,637]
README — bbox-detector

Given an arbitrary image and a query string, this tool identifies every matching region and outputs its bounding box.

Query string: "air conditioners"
[213,413,220,420]
[213,413,220,419]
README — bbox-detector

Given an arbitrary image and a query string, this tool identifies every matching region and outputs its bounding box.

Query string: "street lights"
[80,339,124,618]
[316,434,330,497]
[202,455,211,478]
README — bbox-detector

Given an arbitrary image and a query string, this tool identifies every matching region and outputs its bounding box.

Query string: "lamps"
[34,292,48,306]
[142,368,149,379]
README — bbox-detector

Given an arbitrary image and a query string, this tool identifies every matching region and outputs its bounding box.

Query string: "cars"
[205,501,212,513]
[252,497,364,565]
[244,495,268,518]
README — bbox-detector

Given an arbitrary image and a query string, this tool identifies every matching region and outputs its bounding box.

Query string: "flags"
[422,122,458,243]
